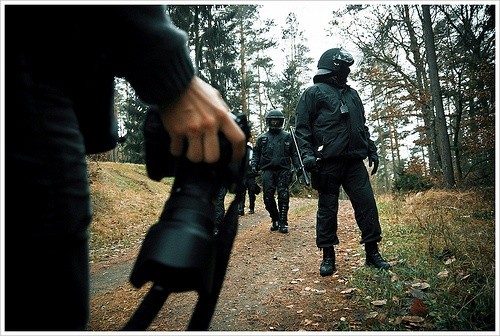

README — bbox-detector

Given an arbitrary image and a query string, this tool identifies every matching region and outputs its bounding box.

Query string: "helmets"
[266,111,285,130]
[313,48,354,83]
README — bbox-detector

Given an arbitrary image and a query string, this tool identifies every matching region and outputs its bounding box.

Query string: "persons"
[236,139,261,216]
[4,4,246,332]
[251,111,303,233]
[294,46,390,277]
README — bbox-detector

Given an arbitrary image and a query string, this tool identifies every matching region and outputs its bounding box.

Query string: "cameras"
[130,110,259,295]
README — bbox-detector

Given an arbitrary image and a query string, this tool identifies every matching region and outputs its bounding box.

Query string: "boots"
[320,246,335,277]
[277,198,288,233]
[365,242,390,270]
[265,197,280,230]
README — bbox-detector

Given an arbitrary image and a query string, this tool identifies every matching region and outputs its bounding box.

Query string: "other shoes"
[238,209,244,216]
[248,208,254,213]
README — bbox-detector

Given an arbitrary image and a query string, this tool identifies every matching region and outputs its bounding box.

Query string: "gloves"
[295,166,303,178]
[251,166,262,178]
[368,154,379,176]
[303,155,320,175]
